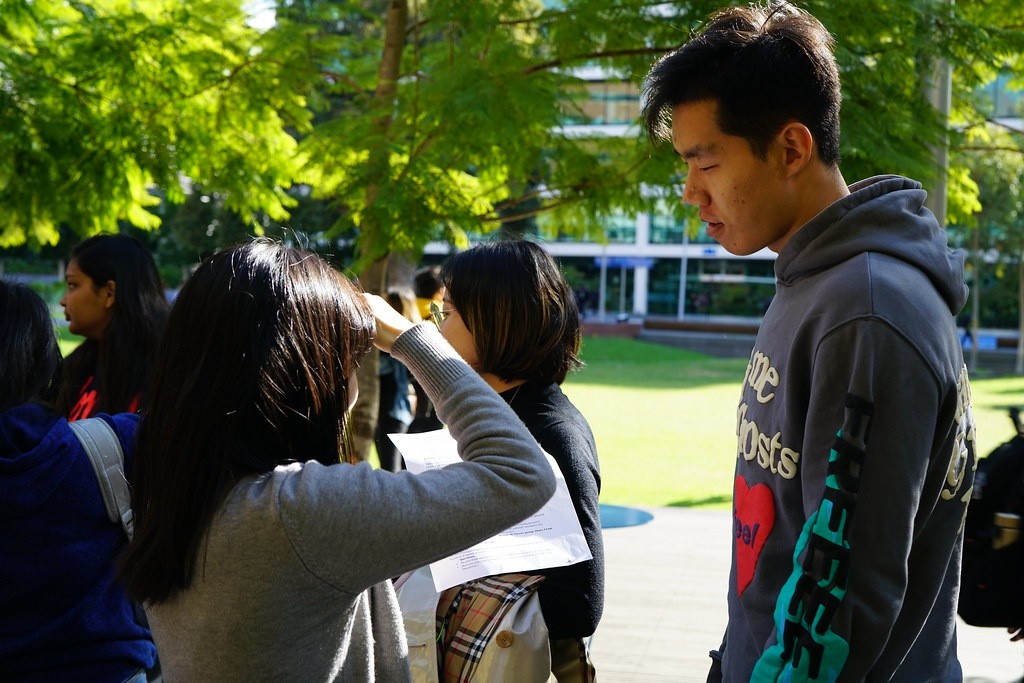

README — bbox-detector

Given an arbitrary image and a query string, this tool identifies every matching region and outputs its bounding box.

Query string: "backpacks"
[958,434,1024,627]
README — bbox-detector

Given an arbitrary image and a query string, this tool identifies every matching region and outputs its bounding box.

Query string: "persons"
[636,5,974,683]
[335,254,454,481]
[0,280,165,683]
[58,233,172,428]
[129,241,557,683]
[398,237,605,683]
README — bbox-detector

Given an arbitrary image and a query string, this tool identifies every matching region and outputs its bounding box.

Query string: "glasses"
[426,302,453,330]
